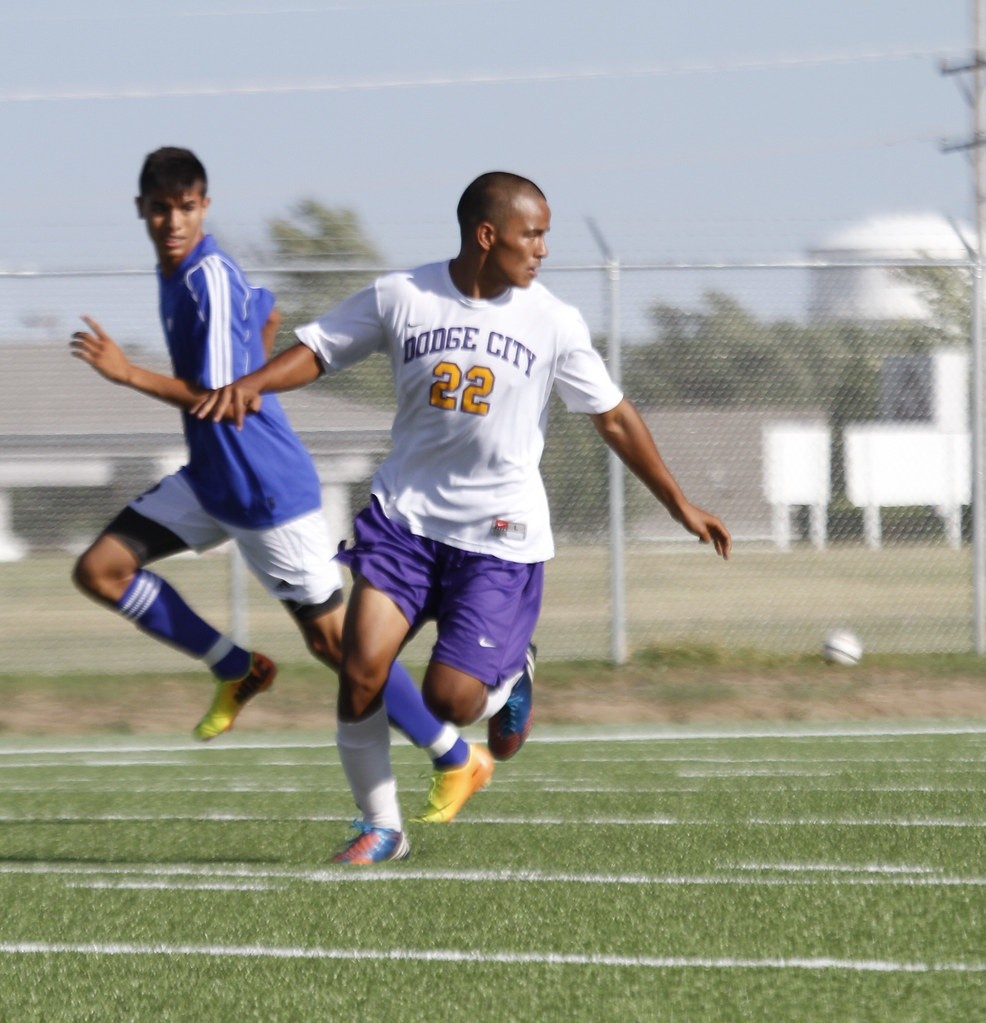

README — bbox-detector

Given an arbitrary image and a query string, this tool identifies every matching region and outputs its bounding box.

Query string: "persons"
[69,145,495,822]
[190,170,732,866]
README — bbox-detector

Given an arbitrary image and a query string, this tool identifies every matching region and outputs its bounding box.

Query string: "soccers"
[825,632,863,667]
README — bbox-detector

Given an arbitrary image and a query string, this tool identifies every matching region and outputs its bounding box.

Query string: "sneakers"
[324,820,412,867]
[488,641,536,761]
[406,743,494,823]
[193,651,277,741]
